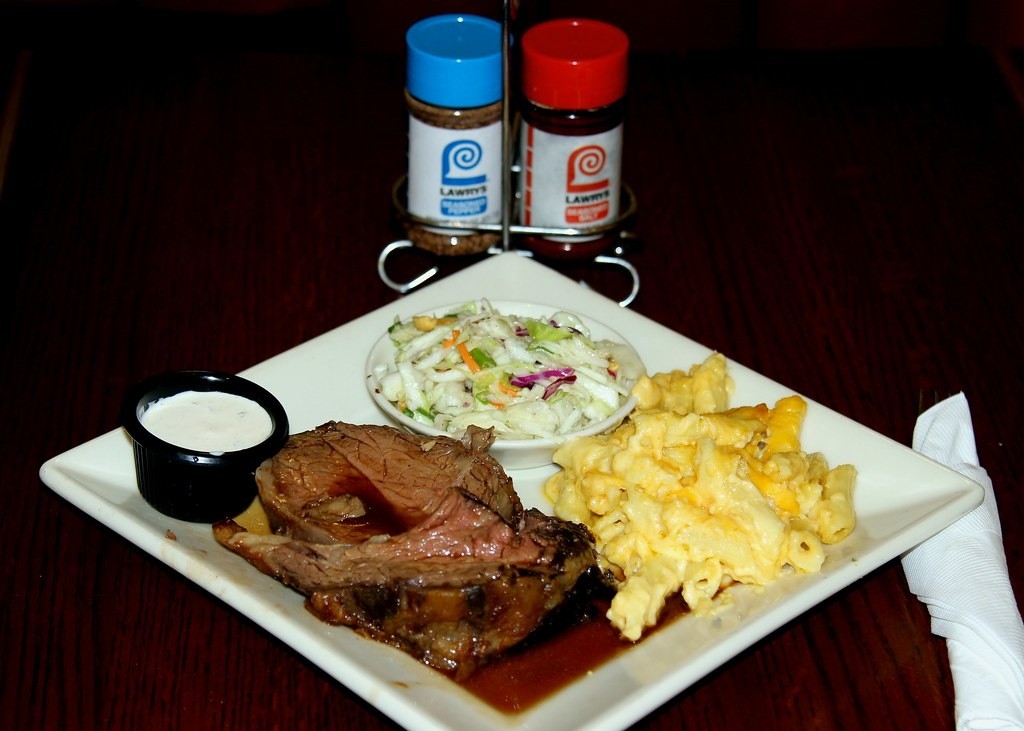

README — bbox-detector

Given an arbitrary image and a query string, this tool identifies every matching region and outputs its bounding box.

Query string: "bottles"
[404,17,514,260]
[520,16,629,266]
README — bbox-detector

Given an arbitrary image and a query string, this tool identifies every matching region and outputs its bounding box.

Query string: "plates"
[39,251,985,731]
[365,300,648,471]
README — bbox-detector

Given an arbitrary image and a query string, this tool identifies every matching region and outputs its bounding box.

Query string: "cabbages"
[375,294,639,443]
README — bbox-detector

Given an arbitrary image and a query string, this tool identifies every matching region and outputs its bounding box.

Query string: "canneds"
[403,14,515,257]
[517,19,627,256]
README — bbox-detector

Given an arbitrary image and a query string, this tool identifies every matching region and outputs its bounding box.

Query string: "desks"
[0,19,1024,731]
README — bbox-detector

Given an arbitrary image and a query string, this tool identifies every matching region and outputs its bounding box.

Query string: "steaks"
[210,420,601,672]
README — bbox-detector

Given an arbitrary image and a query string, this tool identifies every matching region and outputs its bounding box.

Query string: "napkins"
[901,391,1024,731]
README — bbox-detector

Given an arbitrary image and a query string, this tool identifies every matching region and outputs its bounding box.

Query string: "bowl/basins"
[119,369,289,525]
